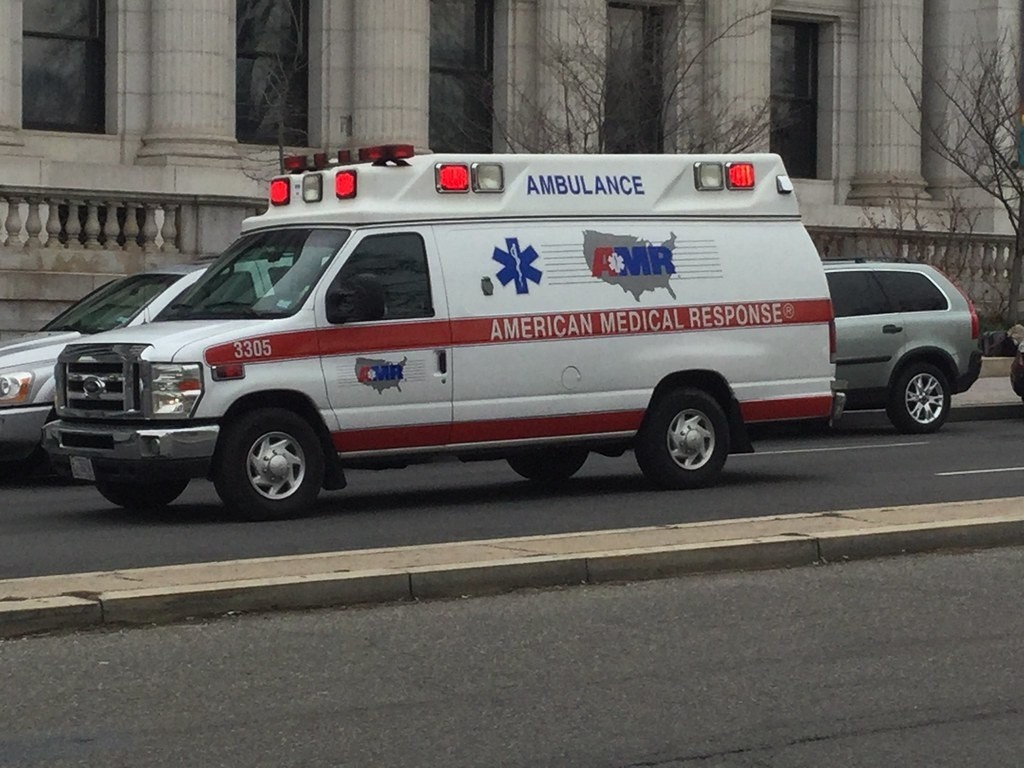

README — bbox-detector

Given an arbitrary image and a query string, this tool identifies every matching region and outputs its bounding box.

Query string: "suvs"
[822,256,983,437]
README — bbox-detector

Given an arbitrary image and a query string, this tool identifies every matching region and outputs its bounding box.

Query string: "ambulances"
[40,142,838,524]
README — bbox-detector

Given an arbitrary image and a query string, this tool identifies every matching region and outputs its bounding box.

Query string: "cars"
[0,256,294,488]
